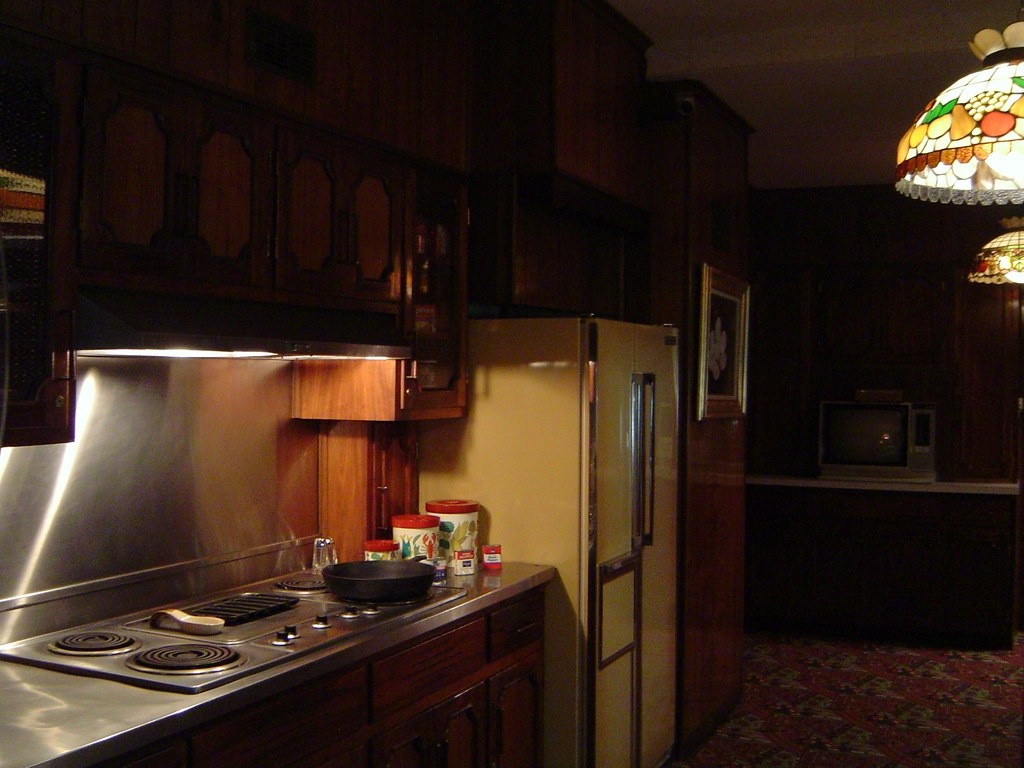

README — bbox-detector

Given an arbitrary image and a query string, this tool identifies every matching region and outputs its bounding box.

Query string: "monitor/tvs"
[818,400,937,484]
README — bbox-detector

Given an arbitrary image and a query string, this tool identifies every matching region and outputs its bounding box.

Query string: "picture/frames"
[693,262,751,423]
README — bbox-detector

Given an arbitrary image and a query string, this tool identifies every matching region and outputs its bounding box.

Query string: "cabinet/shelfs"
[103,586,544,768]
[480,0,658,319]
[0,0,480,450]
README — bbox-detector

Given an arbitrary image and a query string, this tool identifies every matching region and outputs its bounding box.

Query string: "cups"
[414,356,455,390]
[313,539,336,576]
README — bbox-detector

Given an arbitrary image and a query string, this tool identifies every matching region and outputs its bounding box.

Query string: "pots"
[322,555,434,603]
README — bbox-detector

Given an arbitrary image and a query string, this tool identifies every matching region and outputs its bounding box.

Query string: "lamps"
[896,3,1024,206]
[967,211,1024,284]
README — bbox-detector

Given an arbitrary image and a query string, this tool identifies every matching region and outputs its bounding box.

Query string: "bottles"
[413,222,451,303]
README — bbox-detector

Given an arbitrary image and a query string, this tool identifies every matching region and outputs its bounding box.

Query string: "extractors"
[79,295,410,372]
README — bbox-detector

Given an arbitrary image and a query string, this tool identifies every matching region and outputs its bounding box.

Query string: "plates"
[857,388,904,400]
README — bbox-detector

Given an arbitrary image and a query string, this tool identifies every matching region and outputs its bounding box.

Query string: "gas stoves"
[0,561,465,694]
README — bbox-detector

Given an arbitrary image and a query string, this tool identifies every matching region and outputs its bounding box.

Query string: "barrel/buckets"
[364,540,398,562]
[392,514,439,563]
[426,499,479,576]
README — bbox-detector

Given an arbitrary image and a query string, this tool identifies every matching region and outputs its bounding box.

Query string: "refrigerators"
[418,314,679,768]
